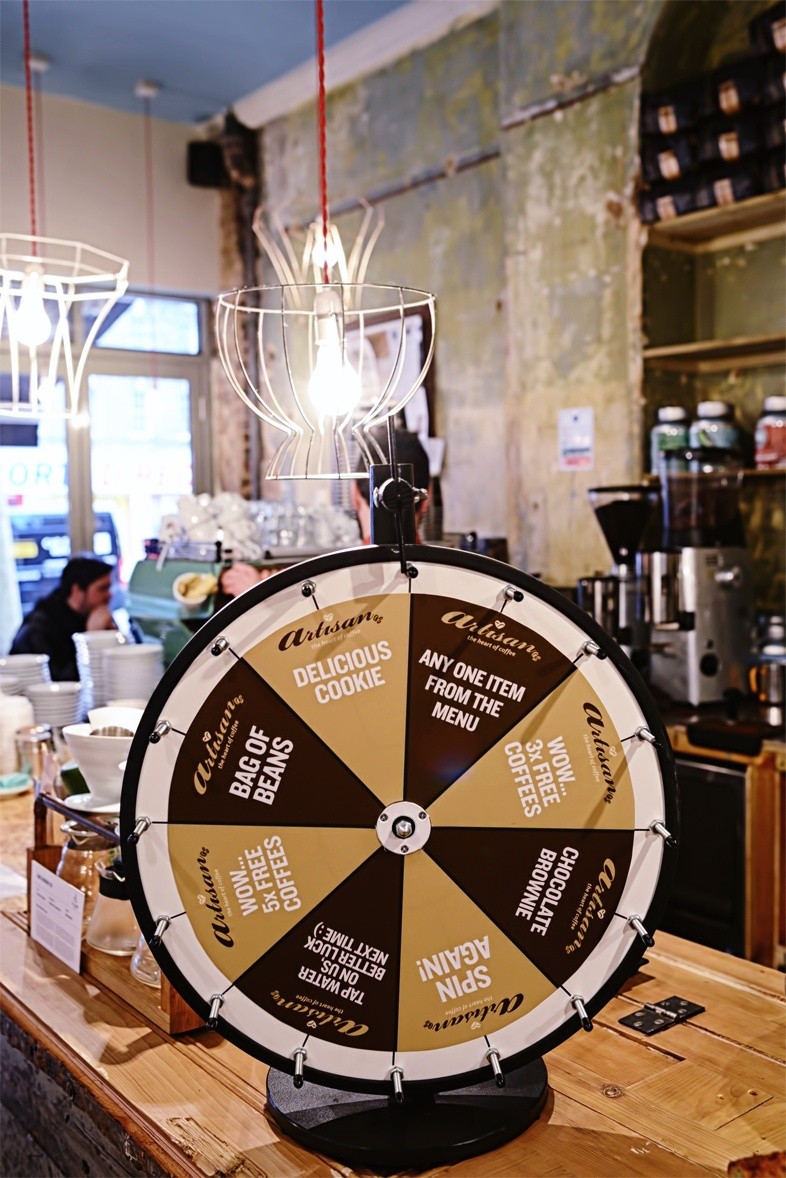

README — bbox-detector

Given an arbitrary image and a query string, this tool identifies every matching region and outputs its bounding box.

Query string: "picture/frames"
[340,302,443,546]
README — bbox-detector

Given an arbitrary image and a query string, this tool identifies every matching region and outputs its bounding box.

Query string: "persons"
[350,428,432,546]
[7,555,118,682]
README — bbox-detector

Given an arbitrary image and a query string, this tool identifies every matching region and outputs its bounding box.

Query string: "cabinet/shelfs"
[641,181,786,487]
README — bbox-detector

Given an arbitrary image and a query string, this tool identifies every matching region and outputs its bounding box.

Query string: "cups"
[172,573,209,611]
[251,500,361,549]
[62,723,135,802]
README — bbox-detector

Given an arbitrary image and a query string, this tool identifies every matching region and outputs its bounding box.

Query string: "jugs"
[57,819,118,940]
[88,855,142,956]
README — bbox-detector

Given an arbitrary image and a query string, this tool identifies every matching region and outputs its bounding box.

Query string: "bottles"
[0,773,35,913]
[15,724,61,793]
[650,407,688,474]
[754,396,786,470]
[687,401,741,473]
[130,932,161,988]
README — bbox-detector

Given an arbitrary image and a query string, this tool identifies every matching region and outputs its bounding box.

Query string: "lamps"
[212,0,439,490]
[0,0,134,423]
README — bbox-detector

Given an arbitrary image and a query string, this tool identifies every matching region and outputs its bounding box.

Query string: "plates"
[103,643,164,708]
[26,682,83,726]
[73,631,123,718]
[0,654,51,696]
[64,793,121,813]
